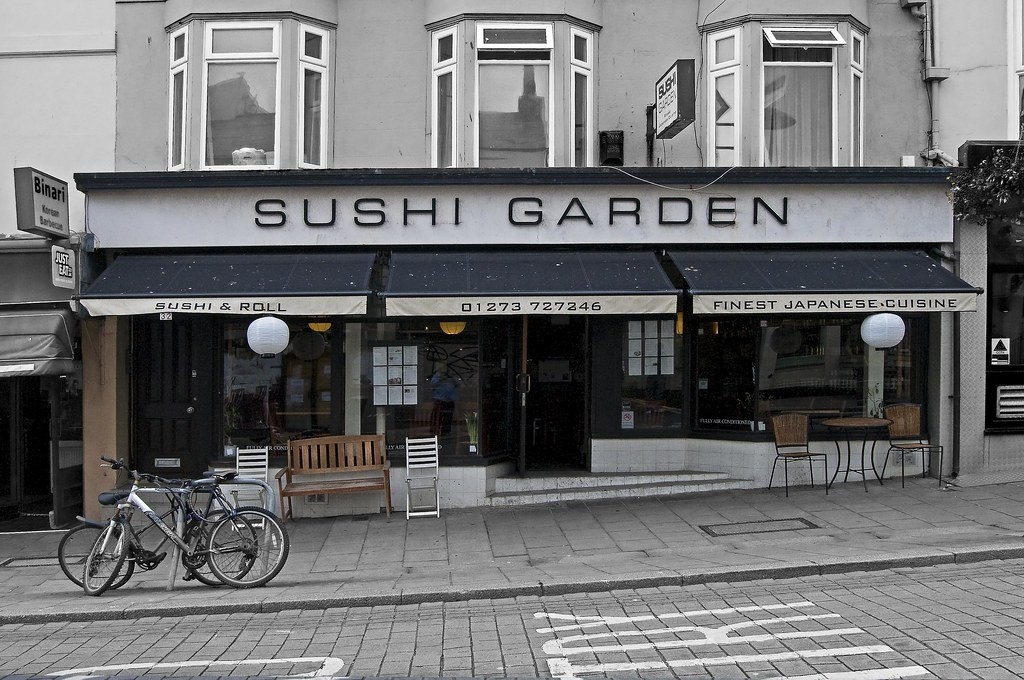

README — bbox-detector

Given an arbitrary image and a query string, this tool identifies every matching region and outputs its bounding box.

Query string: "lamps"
[440,321,467,334]
[308,322,331,331]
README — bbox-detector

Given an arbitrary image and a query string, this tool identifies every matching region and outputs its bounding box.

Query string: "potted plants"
[465,411,478,453]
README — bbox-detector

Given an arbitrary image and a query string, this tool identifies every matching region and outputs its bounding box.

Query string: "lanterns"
[247,317,289,358]
[440,322,467,334]
[860,314,905,351]
[308,323,331,331]
[768,325,803,354]
[293,333,325,362]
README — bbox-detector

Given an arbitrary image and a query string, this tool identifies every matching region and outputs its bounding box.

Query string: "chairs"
[452,399,478,457]
[406,434,440,519]
[410,401,441,447]
[768,413,828,497]
[881,404,943,487]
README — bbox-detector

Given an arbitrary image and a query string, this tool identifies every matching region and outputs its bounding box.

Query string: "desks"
[822,418,894,492]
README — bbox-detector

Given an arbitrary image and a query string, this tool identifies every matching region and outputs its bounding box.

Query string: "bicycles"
[58,458,291,596]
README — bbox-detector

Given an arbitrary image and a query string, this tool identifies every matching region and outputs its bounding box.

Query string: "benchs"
[274,435,392,525]
[229,446,268,531]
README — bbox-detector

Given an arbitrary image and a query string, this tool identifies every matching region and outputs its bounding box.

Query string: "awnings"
[71,252,378,315]
[668,251,984,313]
[0,310,75,377]
[385,252,679,316]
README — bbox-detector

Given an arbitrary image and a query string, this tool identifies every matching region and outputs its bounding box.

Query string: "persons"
[431,372,458,435]
